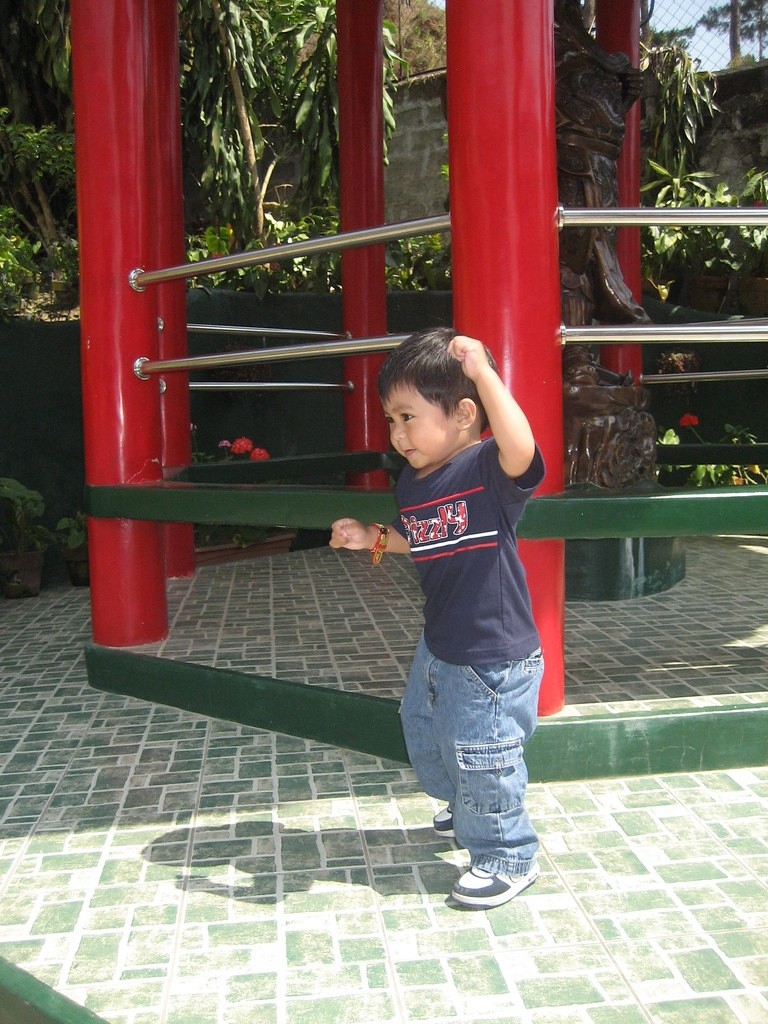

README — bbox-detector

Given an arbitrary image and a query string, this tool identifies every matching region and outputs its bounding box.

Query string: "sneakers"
[433,806,456,838]
[450,858,540,911]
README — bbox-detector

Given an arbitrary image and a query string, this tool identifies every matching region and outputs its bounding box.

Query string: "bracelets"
[367,521,391,566]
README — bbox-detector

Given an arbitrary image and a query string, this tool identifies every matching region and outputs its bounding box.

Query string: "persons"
[327,327,546,912]
[554,0,646,385]
